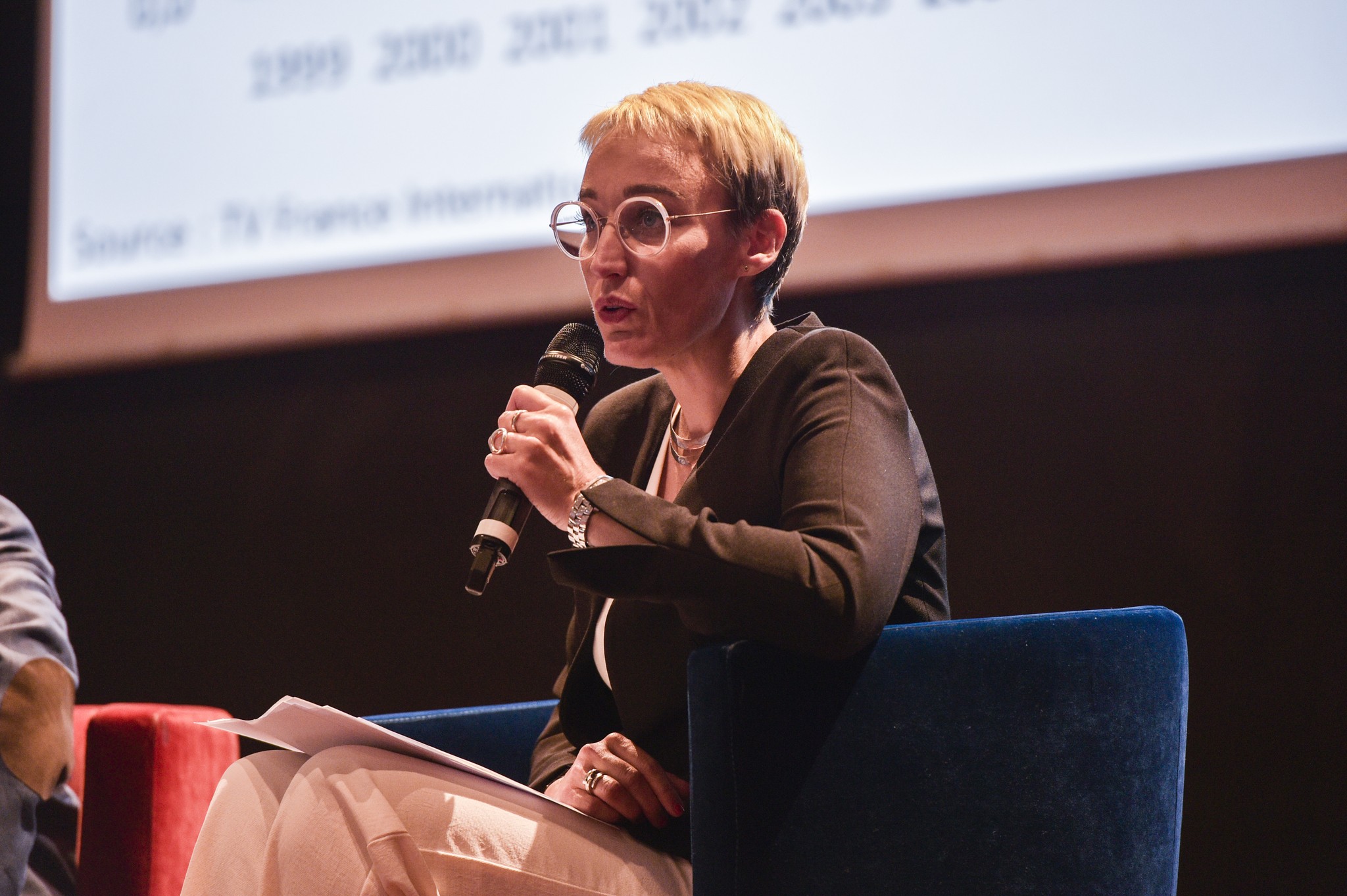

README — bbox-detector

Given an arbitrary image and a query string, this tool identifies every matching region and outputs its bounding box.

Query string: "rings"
[488,427,507,455]
[511,409,529,433]
[582,769,604,796]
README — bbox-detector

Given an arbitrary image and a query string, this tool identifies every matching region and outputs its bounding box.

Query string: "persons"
[179,80,951,896]
[0,493,85,896]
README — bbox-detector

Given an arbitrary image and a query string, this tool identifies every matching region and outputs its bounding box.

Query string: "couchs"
[71,704,240,895]
[358,606,1191,895]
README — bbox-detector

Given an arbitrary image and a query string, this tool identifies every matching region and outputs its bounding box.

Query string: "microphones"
[464,323,605,596]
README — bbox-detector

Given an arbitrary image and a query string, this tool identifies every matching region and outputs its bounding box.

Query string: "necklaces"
[667,399,713,465]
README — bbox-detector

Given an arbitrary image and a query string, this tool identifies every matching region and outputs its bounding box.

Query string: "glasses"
[549,195,739,261]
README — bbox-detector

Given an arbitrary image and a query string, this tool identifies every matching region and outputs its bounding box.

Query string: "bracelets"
[567,473,613,551]
